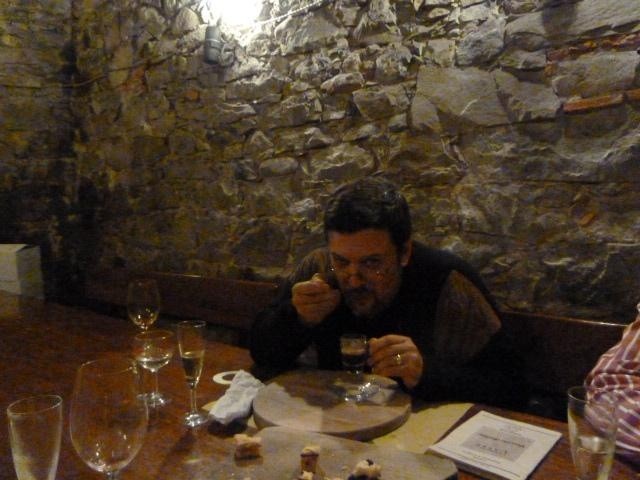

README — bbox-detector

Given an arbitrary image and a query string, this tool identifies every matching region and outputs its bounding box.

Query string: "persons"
[249,175,504,407]
[581,303,640,464]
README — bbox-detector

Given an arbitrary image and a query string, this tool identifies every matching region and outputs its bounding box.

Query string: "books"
[424,410,564,480]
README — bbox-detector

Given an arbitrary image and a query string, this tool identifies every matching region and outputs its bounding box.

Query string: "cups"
[565,385,619,480]
[6,393,64,480]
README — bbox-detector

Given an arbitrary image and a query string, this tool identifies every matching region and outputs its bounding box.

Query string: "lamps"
[203,25,235,68]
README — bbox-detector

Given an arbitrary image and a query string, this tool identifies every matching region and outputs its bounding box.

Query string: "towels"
[206,367,265,426]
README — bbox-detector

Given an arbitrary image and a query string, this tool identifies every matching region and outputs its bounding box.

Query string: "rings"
[395,354,403,365]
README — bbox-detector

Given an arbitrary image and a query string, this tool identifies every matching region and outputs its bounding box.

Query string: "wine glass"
[133,330,174,407]
[338,334,368,403]
[69,358,149,480]
[128,277,162,333]
[176,319,210,426]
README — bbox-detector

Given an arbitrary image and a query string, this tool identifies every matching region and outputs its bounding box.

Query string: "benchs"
[144,272,627,421]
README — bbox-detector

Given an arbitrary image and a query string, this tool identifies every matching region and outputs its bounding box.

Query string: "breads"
[347,459,381,480]
[300,444,320,480]
[233,434,262,458]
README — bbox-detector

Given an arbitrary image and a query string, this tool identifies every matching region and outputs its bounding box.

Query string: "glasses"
[326,253,399,279]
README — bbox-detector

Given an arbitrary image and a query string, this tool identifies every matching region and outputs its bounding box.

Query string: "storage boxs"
[1,242,42,298]
